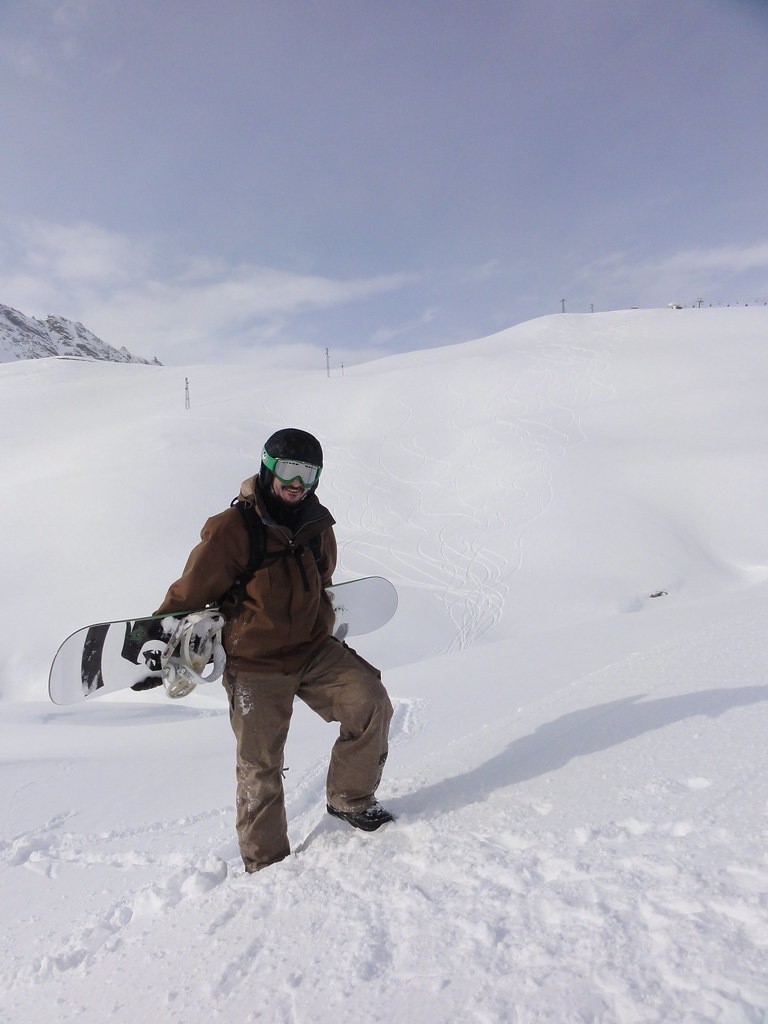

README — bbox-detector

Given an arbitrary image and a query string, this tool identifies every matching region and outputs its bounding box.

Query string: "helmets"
[258,428,323,497]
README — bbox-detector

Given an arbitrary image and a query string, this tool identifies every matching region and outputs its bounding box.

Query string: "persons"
[153,428,395,872]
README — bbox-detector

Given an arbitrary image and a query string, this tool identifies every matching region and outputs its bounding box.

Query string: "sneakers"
[327,803,393,832]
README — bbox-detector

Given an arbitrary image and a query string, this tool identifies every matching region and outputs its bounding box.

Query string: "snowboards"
[46,576,399,707]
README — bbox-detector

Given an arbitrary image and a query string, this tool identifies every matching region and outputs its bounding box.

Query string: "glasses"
[261,447,322,486]
